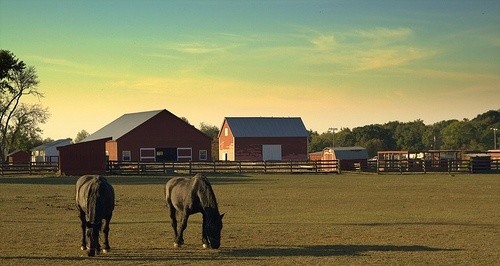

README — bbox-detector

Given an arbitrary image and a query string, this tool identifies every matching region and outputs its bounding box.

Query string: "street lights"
[490,127,498,150]
[329,127,337,147]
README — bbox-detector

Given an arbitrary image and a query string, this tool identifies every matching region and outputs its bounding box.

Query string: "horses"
[164,171,226,249]
[74,174,115,257]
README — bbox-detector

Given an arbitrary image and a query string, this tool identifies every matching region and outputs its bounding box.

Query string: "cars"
[368,156,377,162]
